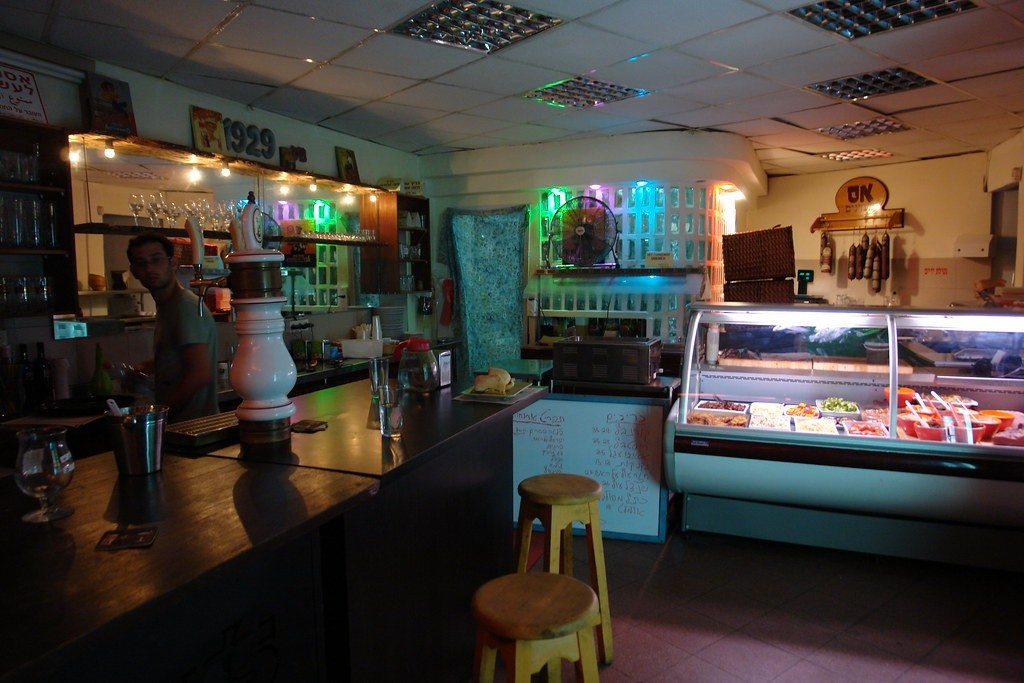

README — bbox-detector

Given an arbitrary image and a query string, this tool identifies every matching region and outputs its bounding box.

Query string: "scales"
[794,269,829,304]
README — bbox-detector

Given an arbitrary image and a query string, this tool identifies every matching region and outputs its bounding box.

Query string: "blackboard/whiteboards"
[510,394,668,542]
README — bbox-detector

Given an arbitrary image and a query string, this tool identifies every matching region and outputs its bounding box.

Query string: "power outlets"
[337,287,347,310]
[289,319,309,334]
[527,296,538,317]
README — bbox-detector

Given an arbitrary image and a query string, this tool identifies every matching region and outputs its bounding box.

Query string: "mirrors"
[68,142,378,316]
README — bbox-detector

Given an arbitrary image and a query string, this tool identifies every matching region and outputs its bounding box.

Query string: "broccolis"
[821,396,858,412]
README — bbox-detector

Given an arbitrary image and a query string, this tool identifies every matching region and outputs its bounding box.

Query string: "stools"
[513,473,614,666]
[470,572,600,683]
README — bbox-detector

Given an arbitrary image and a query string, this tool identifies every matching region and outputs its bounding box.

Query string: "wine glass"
[17,428,76,522]
[129,192,243,232]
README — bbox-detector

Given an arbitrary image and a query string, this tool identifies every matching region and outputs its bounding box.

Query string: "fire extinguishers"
[821,241,832,273]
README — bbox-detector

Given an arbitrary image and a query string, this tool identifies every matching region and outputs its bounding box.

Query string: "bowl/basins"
[898,405,1015,444]
[883,387,915,407]
[88,274,106,291]
[864,342,888,364]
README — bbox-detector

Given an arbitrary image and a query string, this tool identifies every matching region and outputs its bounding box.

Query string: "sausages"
[820,232,890,293]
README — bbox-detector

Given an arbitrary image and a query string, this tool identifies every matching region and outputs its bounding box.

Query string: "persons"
[126,232,221,425]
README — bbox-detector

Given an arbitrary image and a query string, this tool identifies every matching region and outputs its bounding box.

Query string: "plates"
[461,383,533,398]
[913,397,978,409]
[373,307,404,338]
[694,399,889,437]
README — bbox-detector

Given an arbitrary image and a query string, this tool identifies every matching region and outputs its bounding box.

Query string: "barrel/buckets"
[104,405,170,475]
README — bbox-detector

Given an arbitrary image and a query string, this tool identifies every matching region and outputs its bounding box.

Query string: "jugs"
[393,337,440,391]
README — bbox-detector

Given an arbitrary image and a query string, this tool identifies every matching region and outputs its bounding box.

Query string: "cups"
[398,243,423,292]
[1,151,56,315]
[379,385,406,437]
[368,358,389,399]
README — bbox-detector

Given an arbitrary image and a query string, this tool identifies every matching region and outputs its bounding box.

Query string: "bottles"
[418,292,433,315]
[0,345,17,420]
[35,342,52,405]
[372,315,382,341]
[15,344,37,417]
[706,328,719,364]
[52,358,70,399]
[92,342,112,395]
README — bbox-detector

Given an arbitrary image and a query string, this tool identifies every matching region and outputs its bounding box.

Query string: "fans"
[545,196,621,269]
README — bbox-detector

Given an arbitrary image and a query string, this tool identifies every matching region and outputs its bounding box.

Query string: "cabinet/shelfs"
[378,192,432,294]
[0,116,79,317]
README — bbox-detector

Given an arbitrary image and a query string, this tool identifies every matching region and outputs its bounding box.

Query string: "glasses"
[132,254,172,268]
